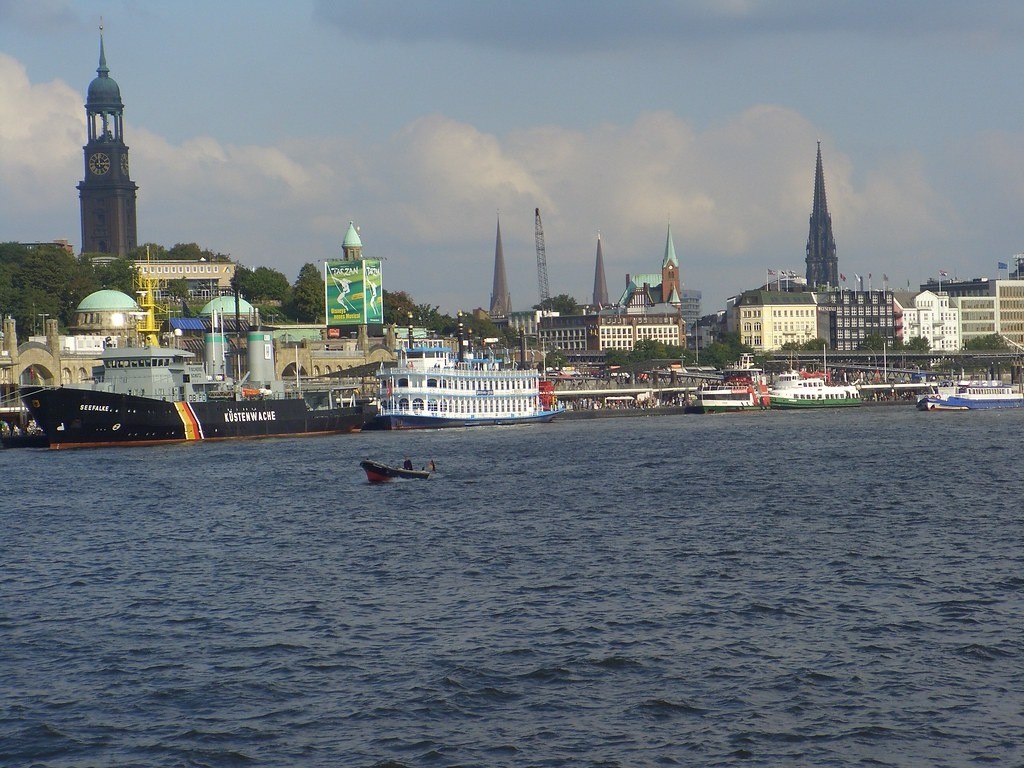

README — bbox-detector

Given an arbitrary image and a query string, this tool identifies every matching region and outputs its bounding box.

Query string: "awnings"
[170,317,206,330]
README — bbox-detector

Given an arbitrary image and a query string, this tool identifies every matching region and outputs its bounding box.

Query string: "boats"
[374,339,568,431]
[915,383,1024,410]
[359,459,432,482]
[770,368,864,408]
[14,346,366,450]
[694,381,772,413]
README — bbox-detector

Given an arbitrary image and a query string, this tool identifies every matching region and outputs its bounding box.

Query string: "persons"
[697,379,751,391]
[539,392,693,409]
[0,422,44,438]
[827,372,966,403]
[454,364,534,372]
[406,361,411,369]
[433,363,438,374]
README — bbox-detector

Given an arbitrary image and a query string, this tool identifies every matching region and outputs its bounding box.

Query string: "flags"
[998,261,1008,269]
[939,267,948,276]
[840,271,911,288]
[387,381,393,397]
[768,268,797,277]
